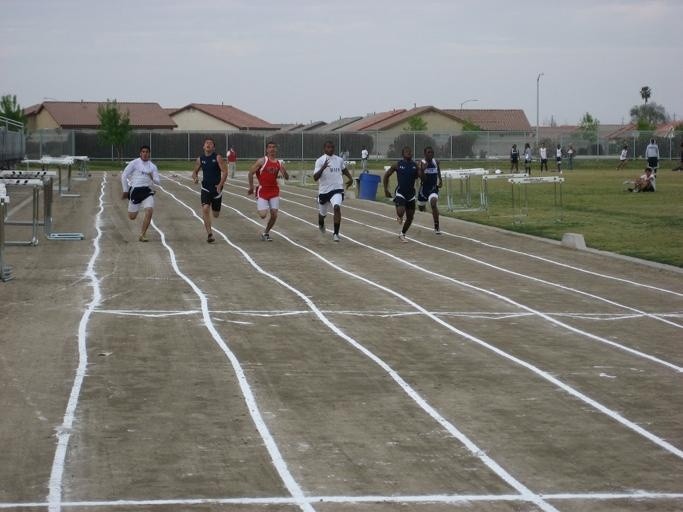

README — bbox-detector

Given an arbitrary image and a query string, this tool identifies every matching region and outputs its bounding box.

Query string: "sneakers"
[139,234,149,242]
[333,234,339,241]
[434,228,440,234]
[262,233,271,241]
[399,232,408,242]
[208,233,214,241]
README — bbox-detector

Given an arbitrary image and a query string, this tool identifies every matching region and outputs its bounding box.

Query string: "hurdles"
[0,155,89,246]
[440,167,565,226]
[0,182,16,283]
[383,165,397,201]
[340,161,356,190]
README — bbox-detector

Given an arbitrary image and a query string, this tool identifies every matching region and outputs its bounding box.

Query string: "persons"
[312,140,353,241]
[247,141,289,242]
[226,143,238,179]
[339,147,349,176]
[615,145,628,170]
[539,143,548,172]
[361,144,369,173]
[192,137,229,242]
[645,138,660,179]
[120,145,161,242]
[523,143,533,175]
[565,142,577,170]
[628,166,657,192]
[384,145,428,242]
[417,145,442,235]
[555,144,563,172]
[510,144,520,174]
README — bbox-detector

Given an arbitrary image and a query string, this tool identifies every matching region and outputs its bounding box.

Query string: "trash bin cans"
[359,175,382,201]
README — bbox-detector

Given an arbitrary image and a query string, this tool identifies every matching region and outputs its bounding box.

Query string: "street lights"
[460,99,480,110]
[536,73,544,147]
